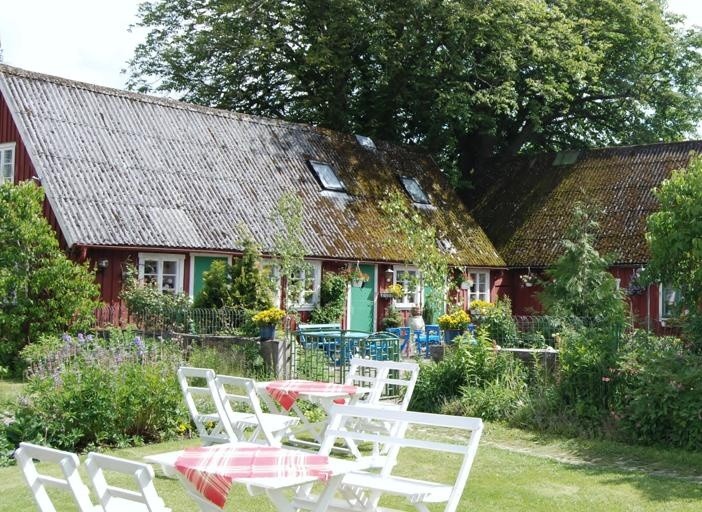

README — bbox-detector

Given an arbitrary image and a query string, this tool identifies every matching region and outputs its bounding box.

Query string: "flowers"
[521,272,538,282]
[251,307,287,328]
[348,268,370,283]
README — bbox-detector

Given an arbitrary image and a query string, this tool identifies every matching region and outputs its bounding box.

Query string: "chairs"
[299,322,443,363]
[14,353,487,511]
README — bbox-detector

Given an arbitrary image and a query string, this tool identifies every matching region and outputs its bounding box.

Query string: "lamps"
[383,264,395,282]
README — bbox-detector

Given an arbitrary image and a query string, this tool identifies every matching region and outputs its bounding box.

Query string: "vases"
[524,282,533,287]
[260,324,275,341]
[353,281,363,288]
